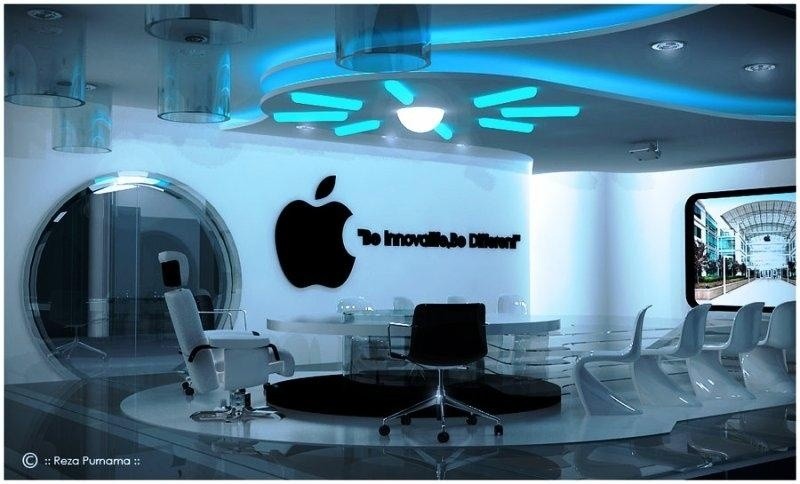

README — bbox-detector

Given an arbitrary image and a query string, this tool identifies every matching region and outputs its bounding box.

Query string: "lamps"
[51,83,111,153]
[158,38,231,123]
[145,5,255,44]
[335,4,432,73]
[4,4,87,109]
[272,80,580,141]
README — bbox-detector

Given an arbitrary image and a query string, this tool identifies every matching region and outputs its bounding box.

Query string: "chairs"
[51,292,110,360]
[740,300,796,401]
[631,303,712,410]
[572,304,654,416]
[335,294,528,369]
[193,288,246,330]
[380,303,502,443]
[685,303,765,403]
[158,250,294,420]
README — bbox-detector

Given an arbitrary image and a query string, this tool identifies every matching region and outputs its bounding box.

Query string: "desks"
[266,309,561,416]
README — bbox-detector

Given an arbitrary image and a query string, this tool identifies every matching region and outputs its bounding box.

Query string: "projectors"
[628,142,661,162]
